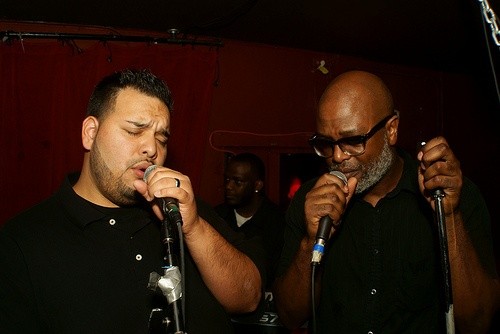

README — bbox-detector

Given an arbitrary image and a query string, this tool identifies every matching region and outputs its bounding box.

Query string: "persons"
[213,153,296,334]
[276,70,500,334]
[0,69,262,334]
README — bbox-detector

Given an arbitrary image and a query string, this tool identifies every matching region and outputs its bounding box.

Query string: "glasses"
[307,111,398,159]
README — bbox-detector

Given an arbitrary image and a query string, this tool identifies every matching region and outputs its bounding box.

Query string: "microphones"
[143,165,183,226]
[311,171,348,264]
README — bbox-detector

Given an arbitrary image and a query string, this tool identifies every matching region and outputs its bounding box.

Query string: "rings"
[175,178,181,187]
